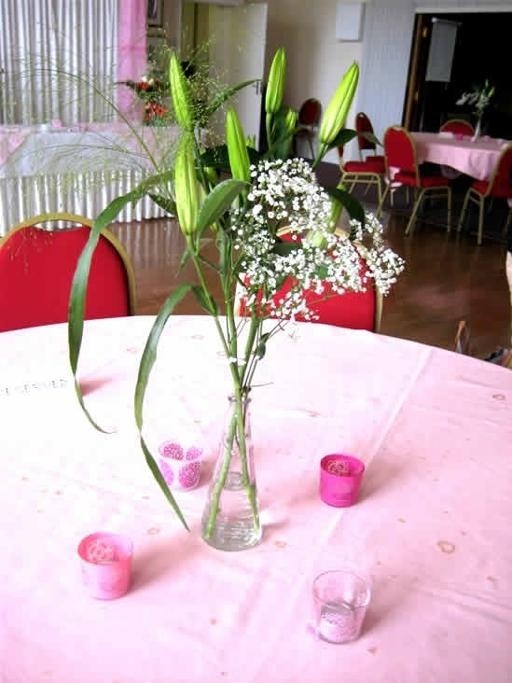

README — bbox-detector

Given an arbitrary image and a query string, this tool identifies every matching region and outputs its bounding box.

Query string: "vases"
[471,119,482,142]
[199,395,264,551]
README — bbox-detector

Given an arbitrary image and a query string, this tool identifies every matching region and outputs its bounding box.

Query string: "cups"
[319,454,364,506]
[313,570,372,644]
[156,437,208,489]
[78,530,134,600]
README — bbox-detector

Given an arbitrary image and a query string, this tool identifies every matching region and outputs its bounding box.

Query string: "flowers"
[457,80,496,120]
[0,16,408,545]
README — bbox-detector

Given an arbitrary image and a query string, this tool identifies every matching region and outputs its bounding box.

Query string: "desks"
[1,123,182,237]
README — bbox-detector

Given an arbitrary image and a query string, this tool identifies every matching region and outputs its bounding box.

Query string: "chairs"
[456,140,511,245]
[0,213,135,332]
[294,98,321,160]
[355,109,395,208]
[335,130,387,217]
[230,222,382,332]
[383,124,453,236]
[440,119,473,178]
